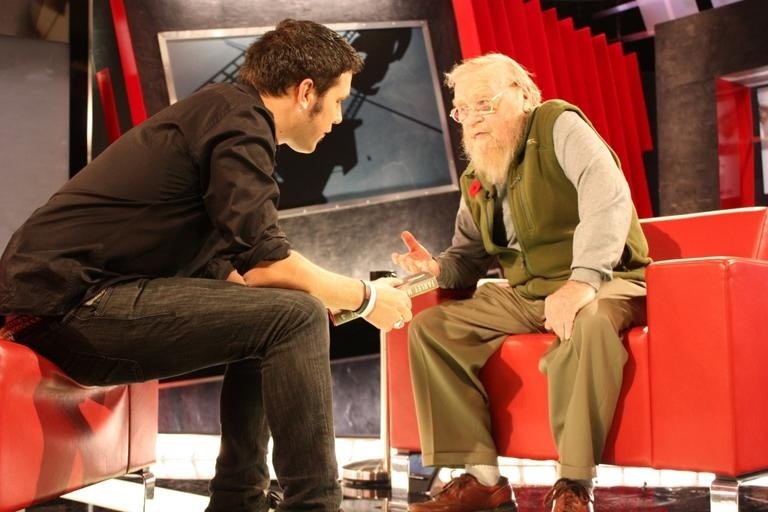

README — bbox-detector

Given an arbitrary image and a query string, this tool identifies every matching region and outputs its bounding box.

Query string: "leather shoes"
[538,477,599,512]
[406,471,518,512]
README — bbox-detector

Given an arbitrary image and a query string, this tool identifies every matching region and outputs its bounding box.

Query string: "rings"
[392,315,405,330]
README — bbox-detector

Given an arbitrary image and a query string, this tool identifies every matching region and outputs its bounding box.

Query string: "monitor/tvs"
[0,1,94,255]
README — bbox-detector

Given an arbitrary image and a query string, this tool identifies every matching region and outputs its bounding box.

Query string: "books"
[327,269,439,327]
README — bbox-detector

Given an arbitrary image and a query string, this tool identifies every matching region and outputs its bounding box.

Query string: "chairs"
[378,206,767,512]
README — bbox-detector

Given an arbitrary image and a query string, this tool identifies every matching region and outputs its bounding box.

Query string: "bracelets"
[351,279,372,315]
[355,279,378,319]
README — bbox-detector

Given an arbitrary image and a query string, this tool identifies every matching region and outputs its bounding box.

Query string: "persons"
[1,18,413,512]
[389,50,658,512]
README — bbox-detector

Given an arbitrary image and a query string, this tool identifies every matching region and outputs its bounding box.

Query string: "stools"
[0,338,160,512]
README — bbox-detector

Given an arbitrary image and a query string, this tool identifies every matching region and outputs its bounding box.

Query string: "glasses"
[448,85,513,123]
